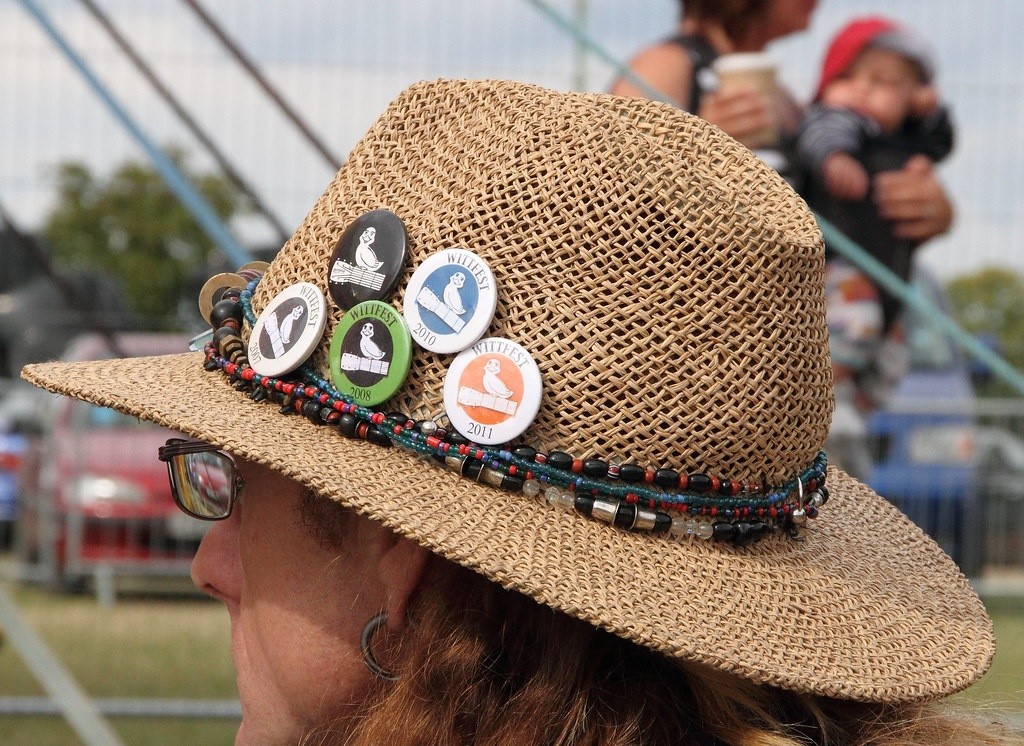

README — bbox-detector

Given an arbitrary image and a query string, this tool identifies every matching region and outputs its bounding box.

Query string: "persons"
[22,77,1024,746]
[607,0,960,238]
[793,19,956,440]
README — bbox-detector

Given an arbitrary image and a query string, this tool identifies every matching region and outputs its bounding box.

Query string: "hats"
[806,15,935,105]
[21,77,996,704]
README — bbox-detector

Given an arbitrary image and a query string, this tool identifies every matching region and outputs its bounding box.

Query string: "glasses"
[156,435,242,521]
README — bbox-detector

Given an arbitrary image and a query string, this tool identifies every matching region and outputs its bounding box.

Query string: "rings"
[923,202,934,218]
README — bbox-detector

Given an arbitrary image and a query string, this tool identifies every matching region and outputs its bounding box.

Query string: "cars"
[31,334,245,569]
[0,395,58,552]
[865,267,1004,576]
